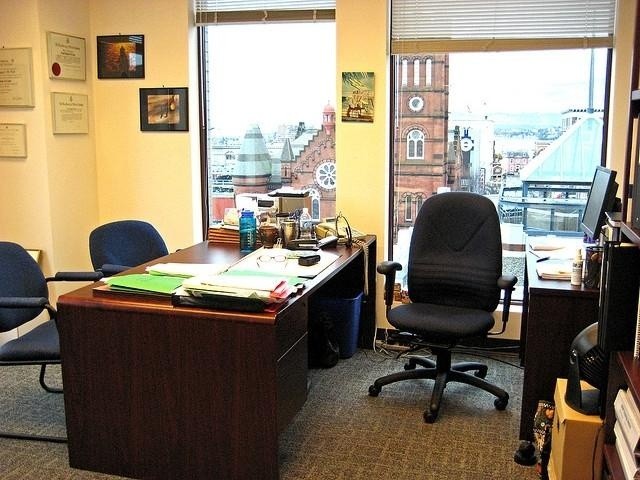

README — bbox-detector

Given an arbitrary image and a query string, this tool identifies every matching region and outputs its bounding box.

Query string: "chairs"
[366,192,518,425]
[1,239,107,446]
[86,217,168,276]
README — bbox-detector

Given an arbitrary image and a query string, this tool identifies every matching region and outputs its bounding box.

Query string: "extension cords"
[375,339,412,352]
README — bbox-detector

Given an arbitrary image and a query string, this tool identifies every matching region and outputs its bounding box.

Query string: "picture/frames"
[95,34,147,81]
[139,85,191,134]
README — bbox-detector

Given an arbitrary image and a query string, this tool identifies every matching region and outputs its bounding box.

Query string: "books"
[532,233,607,283]
[610,386,640,478]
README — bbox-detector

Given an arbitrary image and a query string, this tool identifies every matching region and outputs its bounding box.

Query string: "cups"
[260,213,299,249]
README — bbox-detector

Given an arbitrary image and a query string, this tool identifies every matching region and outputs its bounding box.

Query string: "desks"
[513,237,599,469]
[53,229,378,480]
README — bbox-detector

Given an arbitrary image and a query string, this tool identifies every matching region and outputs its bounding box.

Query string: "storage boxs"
[545,377,606,480]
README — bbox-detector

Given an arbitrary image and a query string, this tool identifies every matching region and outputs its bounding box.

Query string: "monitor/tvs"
[578,166,621,241]
[631,165,640,231]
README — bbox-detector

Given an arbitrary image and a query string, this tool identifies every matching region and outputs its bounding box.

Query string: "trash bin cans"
[315,289,363,359]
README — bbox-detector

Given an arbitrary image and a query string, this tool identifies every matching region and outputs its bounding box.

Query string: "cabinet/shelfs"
[602,0,640,480]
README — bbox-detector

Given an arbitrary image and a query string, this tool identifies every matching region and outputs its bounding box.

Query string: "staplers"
[287,239,319,251]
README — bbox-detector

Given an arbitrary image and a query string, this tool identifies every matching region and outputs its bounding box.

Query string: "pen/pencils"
[537,257,549,262]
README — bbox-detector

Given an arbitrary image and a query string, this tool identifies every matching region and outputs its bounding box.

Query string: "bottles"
[300,208,312,239]
[570,248,583,287]
[239,208,256,253]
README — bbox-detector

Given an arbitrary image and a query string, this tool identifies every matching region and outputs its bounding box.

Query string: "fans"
[563,321,603,417]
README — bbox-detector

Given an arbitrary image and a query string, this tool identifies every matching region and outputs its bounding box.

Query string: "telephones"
[315,222,367,242]
